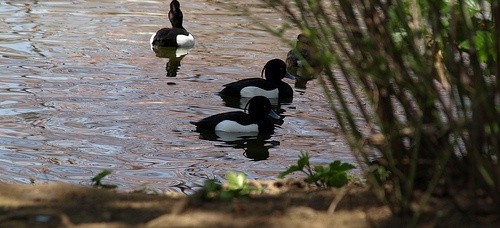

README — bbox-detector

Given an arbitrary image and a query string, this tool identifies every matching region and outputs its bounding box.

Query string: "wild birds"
[190,96,281,133]
[218,59,296,99]
[150,0,195,48]
[285,33,325,81]
